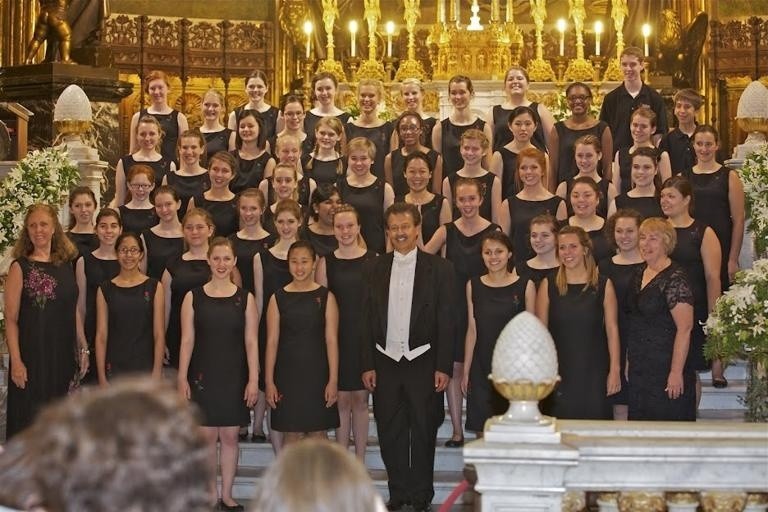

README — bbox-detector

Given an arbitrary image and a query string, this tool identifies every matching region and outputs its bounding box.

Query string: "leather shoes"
[712,375,728,388]
[251,431,267,442]
[220,497,244,511]
[446,435,464,447]
[239,429,248,440]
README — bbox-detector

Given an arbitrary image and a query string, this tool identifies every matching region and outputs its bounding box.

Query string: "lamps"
[730,79,768,159]
[52,81,102,163]
[486,308,563,435]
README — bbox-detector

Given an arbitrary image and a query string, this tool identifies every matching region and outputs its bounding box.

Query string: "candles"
[553,16,570,57]
[385,19,397,61]
[347,17,360,58]
[642,22,653,56]
[301,18,313,60]
[594,18,605,55]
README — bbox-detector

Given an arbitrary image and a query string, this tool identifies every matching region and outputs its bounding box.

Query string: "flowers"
[698,256,768,421]
[546,90,601,124]
[344,98,391,124]
[735,141,767,255]
[0,148,81,256]
[25,264,61,313]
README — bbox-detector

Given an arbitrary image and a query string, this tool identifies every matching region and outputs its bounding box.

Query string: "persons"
[1,373,218,512]
[250,433,392,511]
[359,203,461,511]
[3,71,749,469]
[176,235,262,512]
[598,46,669,160]
[20,1,78,68]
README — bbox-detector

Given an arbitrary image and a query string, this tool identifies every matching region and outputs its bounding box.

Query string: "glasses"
[568,94,591,101]
[118,247,141,255]
[129,183,152,189]
[388,223,411,232]
